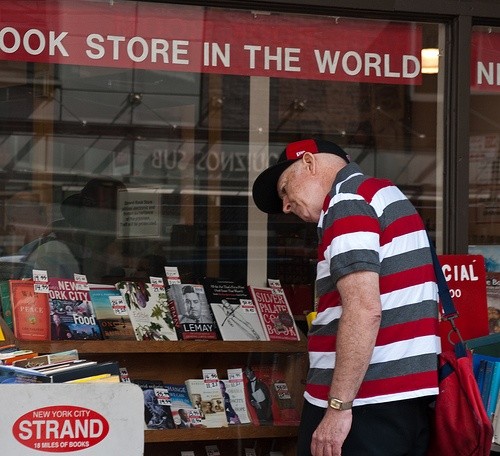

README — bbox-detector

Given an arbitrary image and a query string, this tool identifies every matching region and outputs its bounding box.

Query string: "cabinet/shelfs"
[0,284,312,442]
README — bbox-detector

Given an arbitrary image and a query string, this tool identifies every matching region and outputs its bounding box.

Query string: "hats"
[62,178,130,233]
[253,139,353,216]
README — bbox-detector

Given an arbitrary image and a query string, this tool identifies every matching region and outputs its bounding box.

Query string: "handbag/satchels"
[437,341,493,456]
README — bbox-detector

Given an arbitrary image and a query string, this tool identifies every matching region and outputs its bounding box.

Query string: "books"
[0,268,304,433]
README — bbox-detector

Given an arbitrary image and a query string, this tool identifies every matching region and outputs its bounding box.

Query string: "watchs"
[326,394,353,412]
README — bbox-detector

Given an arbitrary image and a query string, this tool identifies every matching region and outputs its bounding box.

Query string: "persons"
[253,136,446,455]
[20,177,172,282]
[177,286,213,324]
